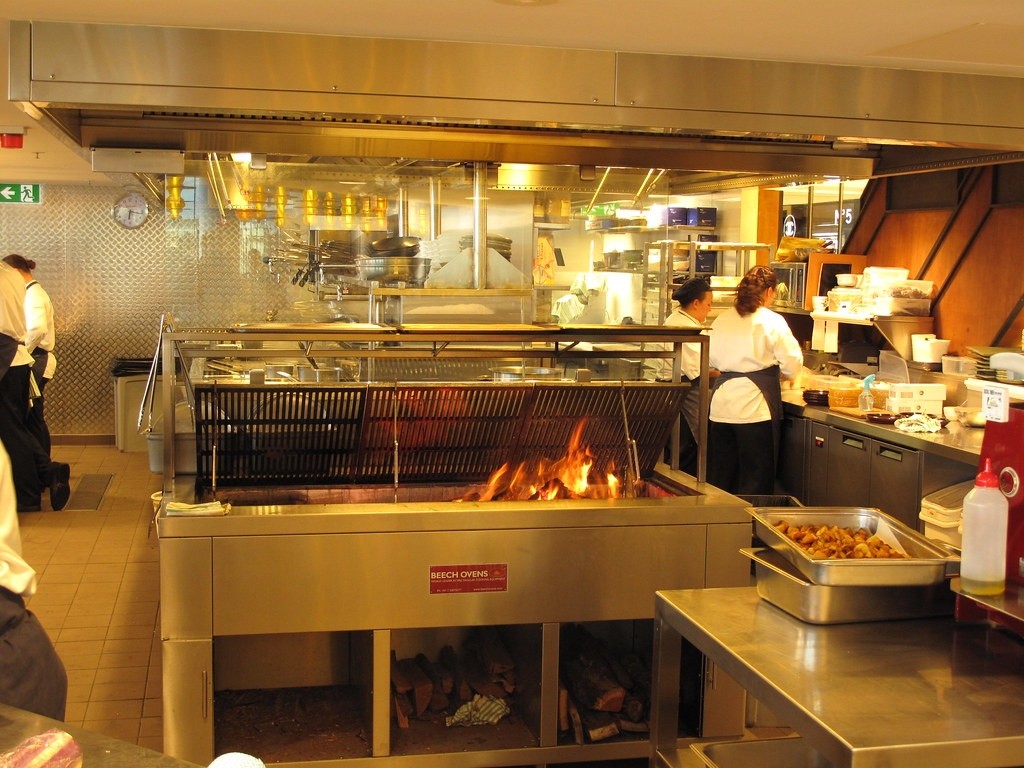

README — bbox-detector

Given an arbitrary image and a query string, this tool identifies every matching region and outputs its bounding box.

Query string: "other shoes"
[50,463,70,511]
[15,502,41,512]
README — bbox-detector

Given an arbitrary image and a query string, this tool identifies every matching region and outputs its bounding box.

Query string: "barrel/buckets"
[146,415,165,473]
[487,365,564,383]
[151,492,163,538]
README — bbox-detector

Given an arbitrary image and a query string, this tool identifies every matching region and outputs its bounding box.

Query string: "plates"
[458,233,512,264]
[153,400,236,433]
[247,393,330,431]
[867,413,902,425]
[966,346,1024,383]
[803,389,829,405]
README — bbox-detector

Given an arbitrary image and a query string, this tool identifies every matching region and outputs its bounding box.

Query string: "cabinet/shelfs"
[827,424,920,531]
[639,240,775,379]
[532,222,571,323]
[653,588,1023,768]
[773,416,807,505]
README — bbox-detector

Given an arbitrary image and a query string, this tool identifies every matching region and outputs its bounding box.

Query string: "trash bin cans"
[919,479,976,549]
[109,356,183,452]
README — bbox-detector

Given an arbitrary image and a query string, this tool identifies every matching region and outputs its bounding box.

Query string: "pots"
[364,236,421,257]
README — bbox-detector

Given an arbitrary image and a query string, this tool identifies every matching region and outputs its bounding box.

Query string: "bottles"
[561,199,571,216]
[960,458,1009,594]
[534,201,544,221]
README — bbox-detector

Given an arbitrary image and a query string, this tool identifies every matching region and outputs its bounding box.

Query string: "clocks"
[111,189,149,230]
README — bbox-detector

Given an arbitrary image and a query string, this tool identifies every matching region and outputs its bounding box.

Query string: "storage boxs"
[687,253,716,272]
[686,207,717,227]
[809,266,976,416]
[662,207,687,226]
[585,220,613,231]
[919,478,977,548]
[146,426,338,473]
[179,342,239,378]
[688,234,718,253]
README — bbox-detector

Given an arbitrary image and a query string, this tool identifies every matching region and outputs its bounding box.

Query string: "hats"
[672,277,710,302]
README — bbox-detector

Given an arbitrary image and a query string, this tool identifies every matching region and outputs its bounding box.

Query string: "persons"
[0,442,67,722]
[708,266,804,496]
[2,254,57,459]
[0,259,70,511]
[655,277,721,477]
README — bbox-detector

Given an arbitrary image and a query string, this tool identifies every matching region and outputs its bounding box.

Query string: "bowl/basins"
[355,258,431,288]
[604,253,620,266]
[625,250,643,262]
